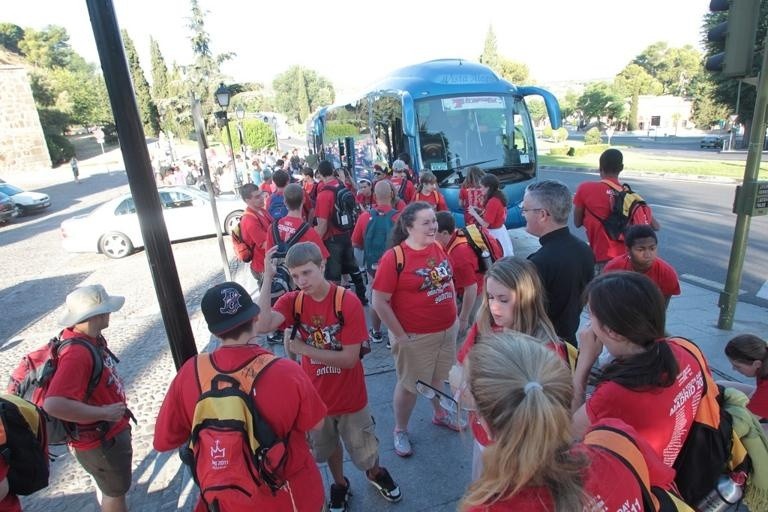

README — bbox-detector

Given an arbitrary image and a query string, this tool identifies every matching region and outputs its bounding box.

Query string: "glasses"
[450,381,472,413]
[519,208,543,217]
[414,377,459,414]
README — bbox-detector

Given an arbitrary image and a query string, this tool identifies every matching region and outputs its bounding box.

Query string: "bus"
[307,59,561,232]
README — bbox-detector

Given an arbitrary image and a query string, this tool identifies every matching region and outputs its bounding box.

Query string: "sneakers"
[393,428,413,456]
[327,475,353,512]
[368,328,383,343]
[266,329,284,344]
[432,409,467,431]
[365,466,402,503]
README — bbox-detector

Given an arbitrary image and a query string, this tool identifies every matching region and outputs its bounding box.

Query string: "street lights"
[216,82,248,193]
[272,116,278,153]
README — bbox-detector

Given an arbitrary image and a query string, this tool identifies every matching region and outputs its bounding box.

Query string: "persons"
[149,147,715,511]
[71,154,80,183]
[725,334,768,425]
[0,285,133,512]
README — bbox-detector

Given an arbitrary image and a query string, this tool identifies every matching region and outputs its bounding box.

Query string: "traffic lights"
[705,1,760,80]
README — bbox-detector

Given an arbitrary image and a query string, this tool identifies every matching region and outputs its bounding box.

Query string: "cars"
[0,183,51,226]
[60,185,269,259]
[701,136,723,148]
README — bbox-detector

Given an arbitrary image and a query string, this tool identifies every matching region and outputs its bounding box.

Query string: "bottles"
[697,472,748,512]
[481,251,493,270]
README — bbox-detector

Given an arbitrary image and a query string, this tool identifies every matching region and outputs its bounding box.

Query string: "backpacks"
[588,180,651,242]
[290,285,370,360]
[669,337,751,512]
[230,179,400,305]
[0,392,58,496]
[582,427,697,512]
[560,336,579,375]
[179,353,288,512]
[5,329,136,446]
[447,224,504,273]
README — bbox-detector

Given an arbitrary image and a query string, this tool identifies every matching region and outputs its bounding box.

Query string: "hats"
[391,159,409,171]
[60,284,125,328]
[201,282,260,336]
